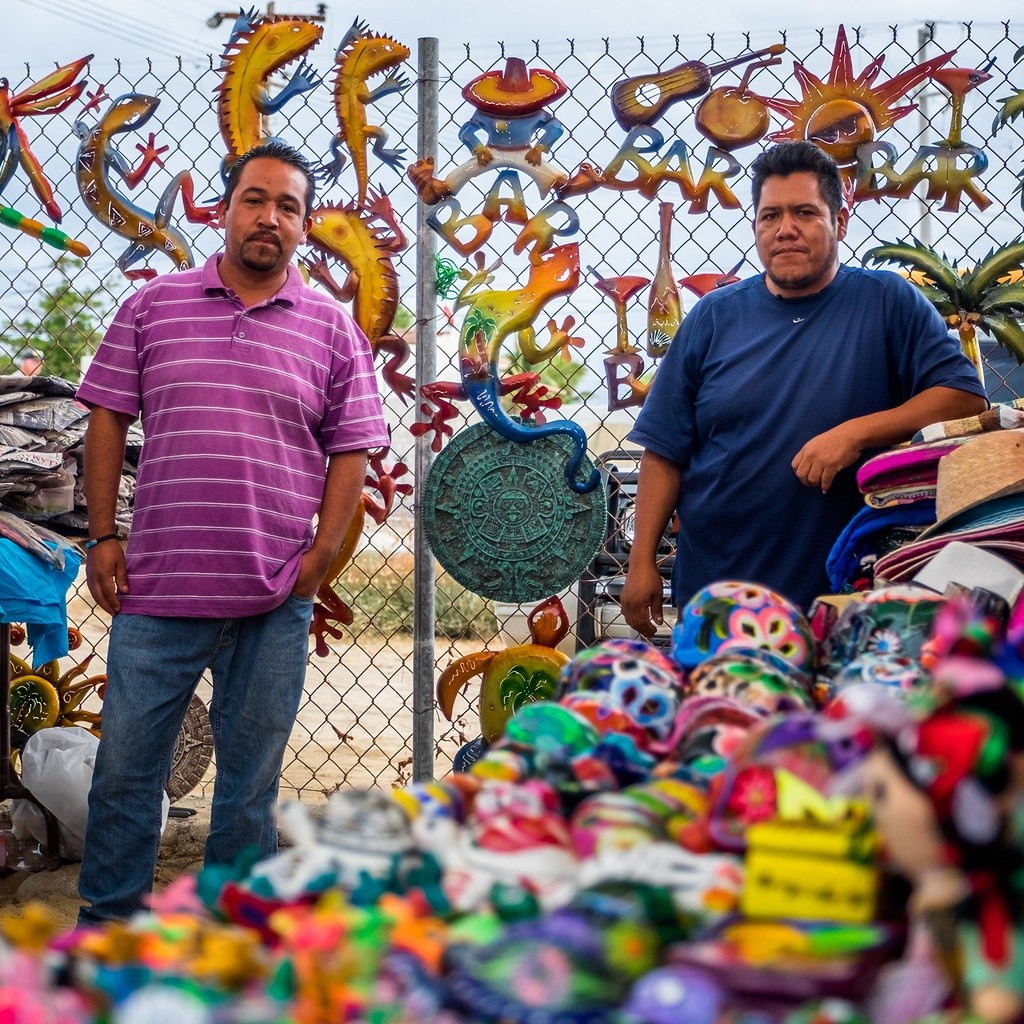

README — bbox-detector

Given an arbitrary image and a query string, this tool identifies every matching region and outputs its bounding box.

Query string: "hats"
[828,431,1024,594]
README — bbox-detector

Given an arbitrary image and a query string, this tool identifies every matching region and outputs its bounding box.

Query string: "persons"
[620,140,991,639]
[75,141,391,930]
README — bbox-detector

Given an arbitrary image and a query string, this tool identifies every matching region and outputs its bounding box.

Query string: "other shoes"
[52,956,73,986]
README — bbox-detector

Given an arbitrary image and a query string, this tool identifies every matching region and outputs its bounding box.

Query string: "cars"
[494,446,681,661]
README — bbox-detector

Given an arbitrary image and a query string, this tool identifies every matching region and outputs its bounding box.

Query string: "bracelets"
[85,534,118,550]
